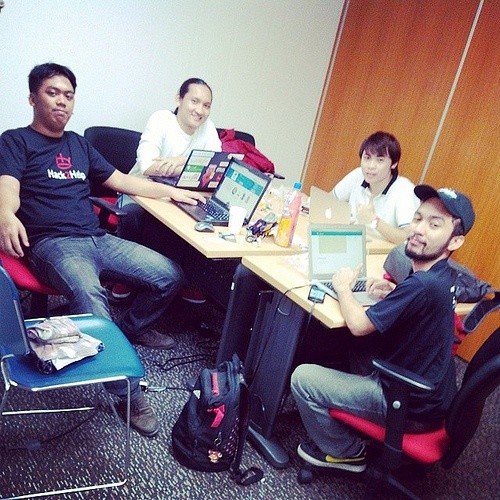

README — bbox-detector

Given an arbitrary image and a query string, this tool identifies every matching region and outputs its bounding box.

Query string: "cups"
[227,205,247,236]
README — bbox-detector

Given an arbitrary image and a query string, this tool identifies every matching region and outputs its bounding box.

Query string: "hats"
[414,182,476,238]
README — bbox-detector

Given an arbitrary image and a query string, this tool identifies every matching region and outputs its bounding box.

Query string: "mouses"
[194,222,215,232]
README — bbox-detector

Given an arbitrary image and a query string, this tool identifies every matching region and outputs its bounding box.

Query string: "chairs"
[0,127,500,500]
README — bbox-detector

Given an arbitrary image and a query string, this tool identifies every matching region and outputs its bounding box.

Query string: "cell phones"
[308,284,327,303]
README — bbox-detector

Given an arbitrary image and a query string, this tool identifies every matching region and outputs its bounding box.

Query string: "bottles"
[275,182,302,248]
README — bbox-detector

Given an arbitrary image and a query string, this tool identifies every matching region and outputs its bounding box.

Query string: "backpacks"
[168,358,265,485]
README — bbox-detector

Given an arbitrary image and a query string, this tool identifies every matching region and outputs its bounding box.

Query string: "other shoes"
[111,279,132,298]
[178,280,207,304]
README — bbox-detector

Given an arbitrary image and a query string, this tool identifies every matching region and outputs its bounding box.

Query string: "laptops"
[309,223,381,307]
[150,149,234,191]
[171,158,271,226]
[309,184,373,242]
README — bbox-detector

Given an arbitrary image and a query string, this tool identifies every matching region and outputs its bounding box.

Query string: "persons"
[0,62,205,439]
[112,78,222,304]
[328,129,421,244]
[291,184,476,473]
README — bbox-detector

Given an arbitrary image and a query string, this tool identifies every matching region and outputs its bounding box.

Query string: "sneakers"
[127,330,175,350]
[297,440,369,473]
[119,389,160,437]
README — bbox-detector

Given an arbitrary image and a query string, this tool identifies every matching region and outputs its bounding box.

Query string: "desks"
[126,184,400,259]
[235,251,399,469]
